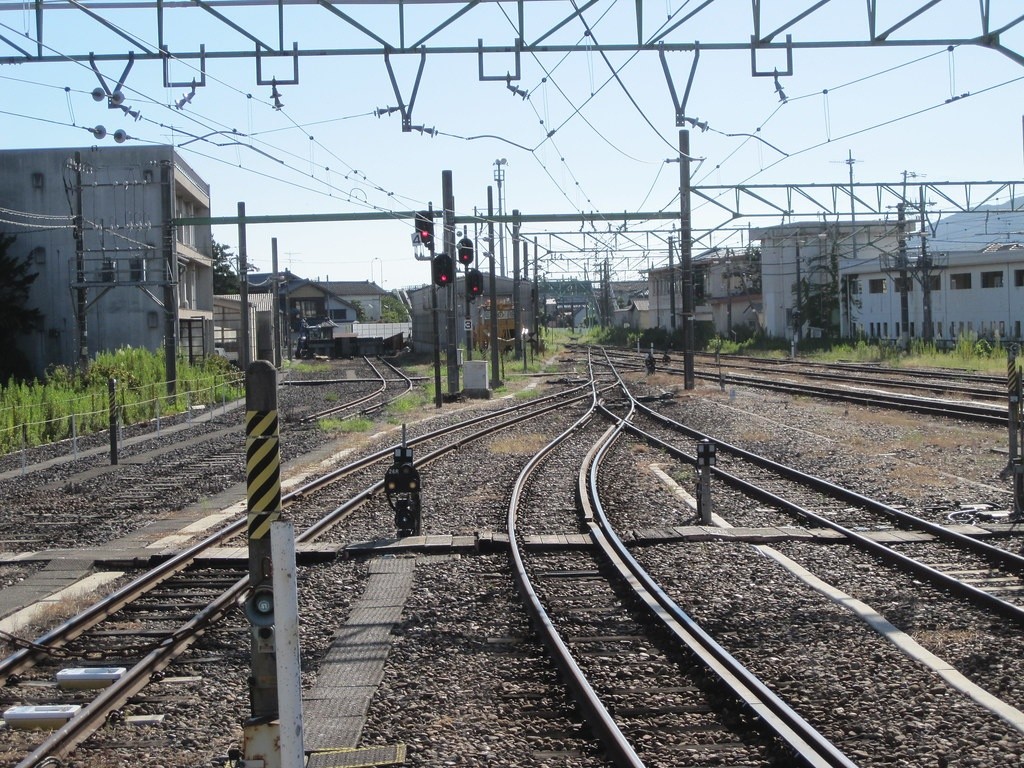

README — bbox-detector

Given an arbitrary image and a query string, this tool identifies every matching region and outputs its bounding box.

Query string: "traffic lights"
[467,269,484,296]
[431,253,454,286]
[458,237,474,265]
[415,210,433,248]
